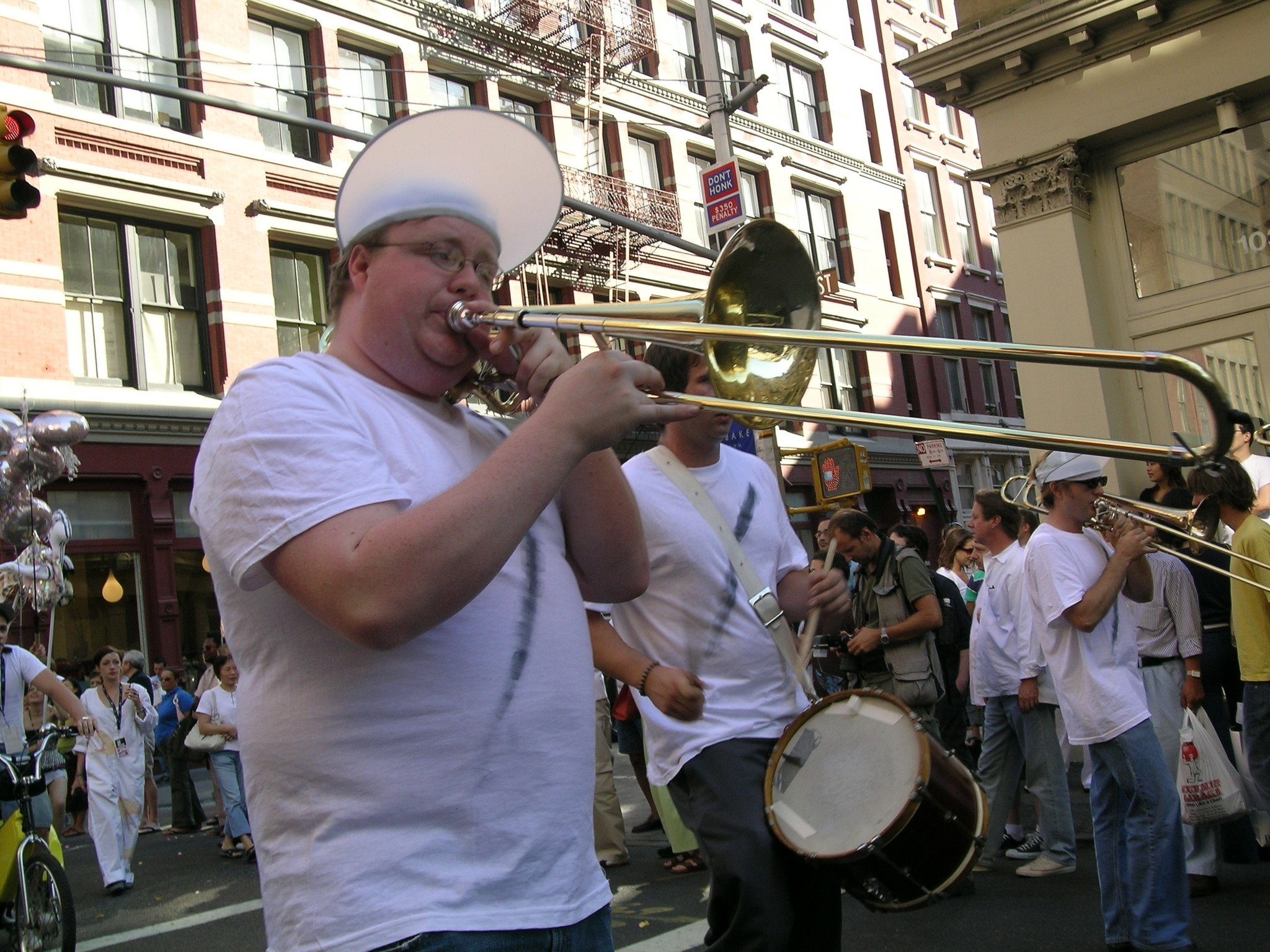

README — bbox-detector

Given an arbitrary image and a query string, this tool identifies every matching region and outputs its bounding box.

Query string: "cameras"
[811,633,863,672]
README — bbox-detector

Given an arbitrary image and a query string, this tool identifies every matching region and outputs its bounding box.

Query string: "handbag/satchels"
[1229,701,1270,847]
[1176,704,1253,824]
[184,689,226,751]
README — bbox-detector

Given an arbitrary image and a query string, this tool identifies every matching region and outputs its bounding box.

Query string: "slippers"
[61,816,257,896]
[664,852,694,868]
[672,856,707,874]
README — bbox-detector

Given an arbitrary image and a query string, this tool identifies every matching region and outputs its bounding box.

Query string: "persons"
[575,337,849,949]
[0,409,1270,952]
[188,104,662,952]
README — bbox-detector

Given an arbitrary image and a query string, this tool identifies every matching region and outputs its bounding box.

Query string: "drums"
[765,688,989,911]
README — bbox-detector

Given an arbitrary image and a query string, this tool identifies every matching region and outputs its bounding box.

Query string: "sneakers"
[997,827,1026,854]
[1016,855,1076,877]
[1005,831,1043,859]
[972,863,993,872]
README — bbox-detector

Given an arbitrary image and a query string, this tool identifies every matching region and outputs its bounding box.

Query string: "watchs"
[880,628,889,645]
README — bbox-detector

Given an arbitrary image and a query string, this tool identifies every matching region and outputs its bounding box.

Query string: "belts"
[1138,655,1175,668]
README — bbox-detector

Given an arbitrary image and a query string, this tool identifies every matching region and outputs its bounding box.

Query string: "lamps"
[101,566,124,603]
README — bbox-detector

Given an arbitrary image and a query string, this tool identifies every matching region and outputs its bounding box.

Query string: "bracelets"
[637,660,660,697]
[1186,670,1200,678]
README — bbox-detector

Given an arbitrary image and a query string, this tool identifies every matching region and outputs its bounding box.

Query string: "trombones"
[445,218,1232,464]
[1001,472,1270,593]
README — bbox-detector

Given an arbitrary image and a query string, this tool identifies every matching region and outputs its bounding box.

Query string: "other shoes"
[9,928,44,952]
[657,845,675,859]
[632,814,661,833]
[1187,872,1218,899]
[599,860,630,867]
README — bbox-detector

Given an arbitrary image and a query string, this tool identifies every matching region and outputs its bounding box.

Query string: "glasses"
[959,546,973,554]
[0,625,7,633]
[814,529,829,538]
[344,241,505,291]
[200,644,217,650]
[159,677,175,681]
[1071,476,1107,489]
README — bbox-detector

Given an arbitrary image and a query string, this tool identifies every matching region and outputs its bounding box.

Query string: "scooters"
[0,721,97,952]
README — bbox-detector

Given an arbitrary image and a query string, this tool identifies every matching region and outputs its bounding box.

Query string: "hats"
[0,603,15,623]
[1035,450,1110,484]
[334,105,564,276]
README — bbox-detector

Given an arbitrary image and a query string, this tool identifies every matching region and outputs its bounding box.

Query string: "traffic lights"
[0,104,41,220]
[811,438,872,501]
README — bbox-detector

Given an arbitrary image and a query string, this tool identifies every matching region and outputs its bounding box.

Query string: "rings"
[82,716,89,720]
